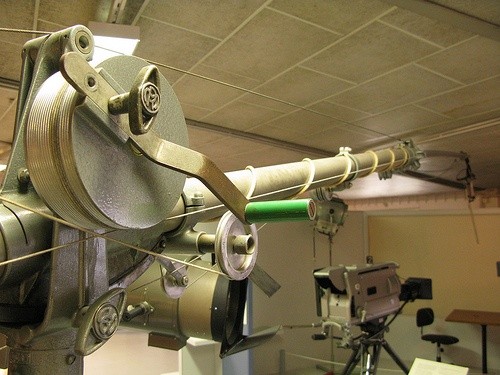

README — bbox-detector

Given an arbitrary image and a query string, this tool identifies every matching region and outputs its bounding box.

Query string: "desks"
[445,309,500,373]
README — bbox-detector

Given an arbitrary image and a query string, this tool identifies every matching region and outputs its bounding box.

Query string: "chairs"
[416,307,460,362]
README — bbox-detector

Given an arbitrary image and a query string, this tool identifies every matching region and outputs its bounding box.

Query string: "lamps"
[87,21,141,64]
